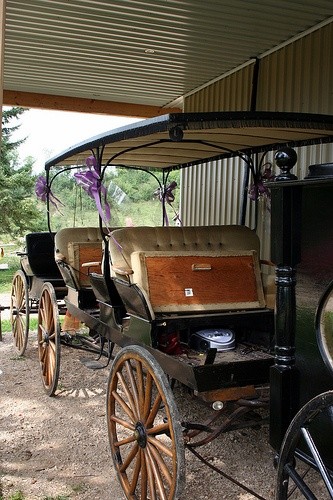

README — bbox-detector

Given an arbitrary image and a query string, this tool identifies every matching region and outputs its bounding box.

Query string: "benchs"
[27,227,278,321]
